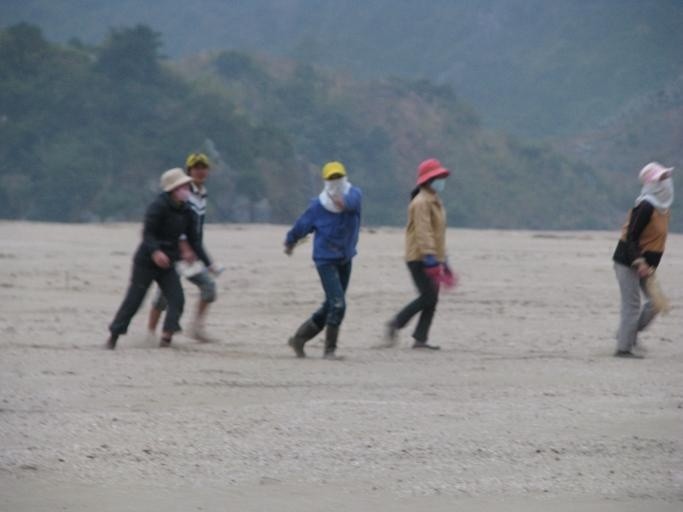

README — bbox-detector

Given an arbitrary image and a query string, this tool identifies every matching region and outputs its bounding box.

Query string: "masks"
[429,178,445,192]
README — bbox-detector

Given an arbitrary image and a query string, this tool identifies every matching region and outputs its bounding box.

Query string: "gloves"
[425,265,454,286]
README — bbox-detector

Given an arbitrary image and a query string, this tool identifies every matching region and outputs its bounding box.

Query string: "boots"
[286,318,346,361]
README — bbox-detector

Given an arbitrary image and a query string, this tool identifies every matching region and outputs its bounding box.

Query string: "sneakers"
[612,350,643,358]
[412,341,440,350]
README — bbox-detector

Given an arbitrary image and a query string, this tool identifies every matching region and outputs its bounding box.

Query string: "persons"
[145,153,220,343]
[284,161,362,360]
[383,159,456,351]
[613,161,674,358]
[103,167,218,349]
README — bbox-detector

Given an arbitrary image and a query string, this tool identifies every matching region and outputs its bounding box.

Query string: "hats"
[159,168,194,193]
[184,153,209,169]
[321,162,346,180]
[638,162,674,186]
[415,159,449,186]
